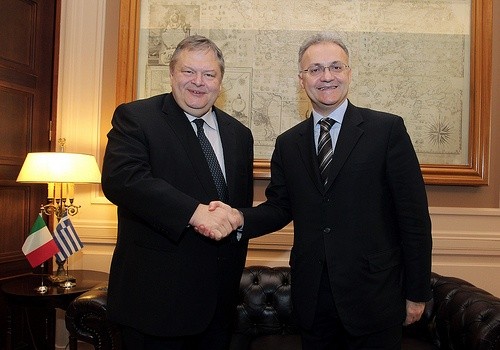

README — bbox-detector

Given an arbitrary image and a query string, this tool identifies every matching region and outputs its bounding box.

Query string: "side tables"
[0,270,109,350]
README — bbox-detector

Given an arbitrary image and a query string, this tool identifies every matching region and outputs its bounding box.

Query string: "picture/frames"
[116,0,494,186]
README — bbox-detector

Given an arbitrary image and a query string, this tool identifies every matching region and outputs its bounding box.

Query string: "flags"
[21,212,60,267]
[52,216,84,263]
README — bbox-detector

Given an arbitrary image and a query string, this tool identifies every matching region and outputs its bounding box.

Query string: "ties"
[192,118,228,204]
[317,117,337,190]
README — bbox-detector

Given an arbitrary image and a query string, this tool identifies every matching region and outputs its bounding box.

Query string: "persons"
[195,33,433,350]
[101,35,253,350]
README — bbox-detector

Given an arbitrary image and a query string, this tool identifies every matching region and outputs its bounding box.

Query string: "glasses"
[299,62,350,76]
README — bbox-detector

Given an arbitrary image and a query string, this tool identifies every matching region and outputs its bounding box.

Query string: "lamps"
[16,138,101,285]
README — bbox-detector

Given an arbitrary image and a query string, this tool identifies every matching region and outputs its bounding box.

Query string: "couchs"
[65,265,500,350]
